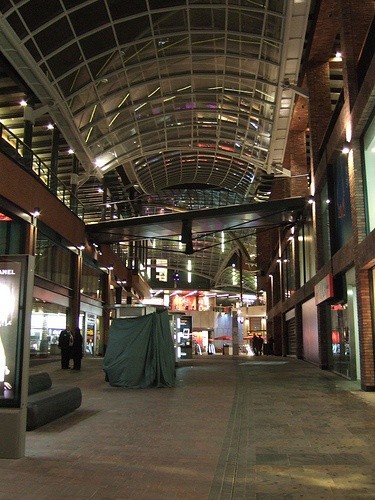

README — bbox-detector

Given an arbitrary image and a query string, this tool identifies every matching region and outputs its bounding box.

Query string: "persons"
[250,333,258,356]
[58,324,73,370]
[252,335,256,356]
[72,328,83,370]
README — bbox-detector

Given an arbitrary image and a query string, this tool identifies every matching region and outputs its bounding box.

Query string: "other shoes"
[72,368,80,370]
[62,366,70,369]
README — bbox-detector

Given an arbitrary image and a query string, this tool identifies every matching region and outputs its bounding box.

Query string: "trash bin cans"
[262,344,272,355]
[223,343,229,354]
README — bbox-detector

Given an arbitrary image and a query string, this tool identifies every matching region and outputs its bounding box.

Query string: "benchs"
[25,371,83,432]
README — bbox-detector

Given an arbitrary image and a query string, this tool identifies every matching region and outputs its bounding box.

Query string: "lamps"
[342,141,352,155]
[335,47,342,58]
[283,77,289,85]
[107,264,114,271]
[308,195,315,205]
[121,278,127,284]
[88,239,103,256]
[33,206,41,219]
[261,288,264,292]
[269,271,272,277]
[79,241,86,251]
[289,233,294,240]
